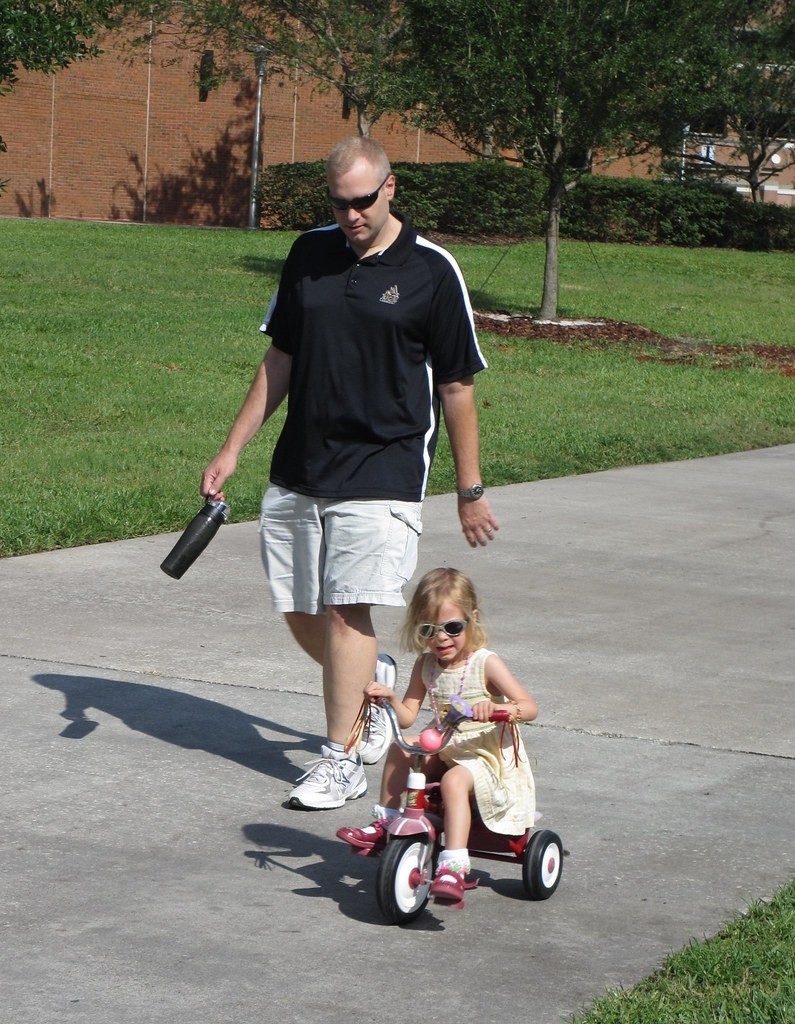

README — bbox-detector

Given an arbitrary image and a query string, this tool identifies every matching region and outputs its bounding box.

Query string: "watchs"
[457,484,484,500]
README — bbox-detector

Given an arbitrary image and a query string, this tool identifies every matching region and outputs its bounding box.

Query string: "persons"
[336,569,536,901]
[200,138,499,810]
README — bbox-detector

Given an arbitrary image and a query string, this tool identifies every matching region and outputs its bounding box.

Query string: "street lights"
[246,42,277,230]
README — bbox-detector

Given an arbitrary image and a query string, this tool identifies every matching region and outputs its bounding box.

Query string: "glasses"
[315,177,391,211]
[415,620,467,640]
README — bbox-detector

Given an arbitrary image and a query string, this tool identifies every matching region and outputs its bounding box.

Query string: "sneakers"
[357,654,395,766]
[287,745,367,808]
[434,869,478,899]
[337,822,391,850]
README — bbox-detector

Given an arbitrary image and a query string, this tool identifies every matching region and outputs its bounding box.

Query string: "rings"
[488,529,492,532]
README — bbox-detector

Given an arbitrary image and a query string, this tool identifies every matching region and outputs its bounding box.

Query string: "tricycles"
[346,694,563,926]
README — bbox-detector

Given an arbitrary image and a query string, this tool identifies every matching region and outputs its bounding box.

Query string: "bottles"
[160,501,227,580]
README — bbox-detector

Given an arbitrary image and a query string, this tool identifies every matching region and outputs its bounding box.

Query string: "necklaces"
[429,652,469,731]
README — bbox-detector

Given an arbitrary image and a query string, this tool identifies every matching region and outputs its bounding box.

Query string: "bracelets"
[505,700,521,723]
